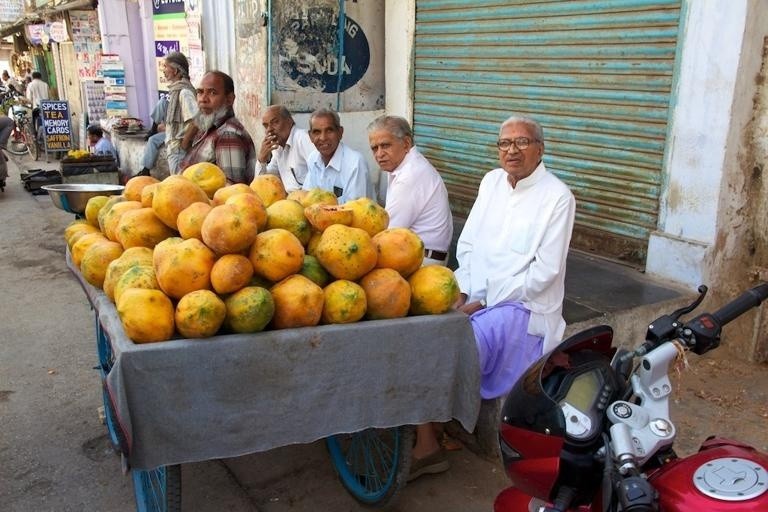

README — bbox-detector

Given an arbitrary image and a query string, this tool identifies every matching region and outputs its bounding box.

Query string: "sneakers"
[382,448,451,484]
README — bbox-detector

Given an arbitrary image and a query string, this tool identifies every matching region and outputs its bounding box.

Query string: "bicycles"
[1,93,39,160]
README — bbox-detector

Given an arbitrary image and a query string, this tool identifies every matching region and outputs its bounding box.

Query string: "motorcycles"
[490,276,768,512]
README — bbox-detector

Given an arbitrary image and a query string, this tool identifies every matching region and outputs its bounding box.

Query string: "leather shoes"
[131,170,150,178]
[143,129,159,141]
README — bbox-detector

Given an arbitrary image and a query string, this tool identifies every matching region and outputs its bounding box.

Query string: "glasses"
[496,137,540,152]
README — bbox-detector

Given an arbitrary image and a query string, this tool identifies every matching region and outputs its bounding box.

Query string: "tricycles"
[63,176,478,510]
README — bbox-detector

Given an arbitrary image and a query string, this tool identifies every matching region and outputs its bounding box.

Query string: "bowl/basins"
[38,181,124,213]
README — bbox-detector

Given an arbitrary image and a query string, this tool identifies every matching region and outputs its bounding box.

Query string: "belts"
[422,247,447,262]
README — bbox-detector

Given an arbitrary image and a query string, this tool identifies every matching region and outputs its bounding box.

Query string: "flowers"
[65,145,89,163]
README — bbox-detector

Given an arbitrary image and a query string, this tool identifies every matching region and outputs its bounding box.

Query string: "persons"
[176,71,255,182]
[302,107,378,206]
[253,104,320,194]
[1,68,54,161]
[395,116,578,487]
[84,126,119,162]
[162,51,202,175]
[369,115,453,279]
[133,87,169,176]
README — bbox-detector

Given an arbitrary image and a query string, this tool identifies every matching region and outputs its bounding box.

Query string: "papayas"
[62,161,459,345]
